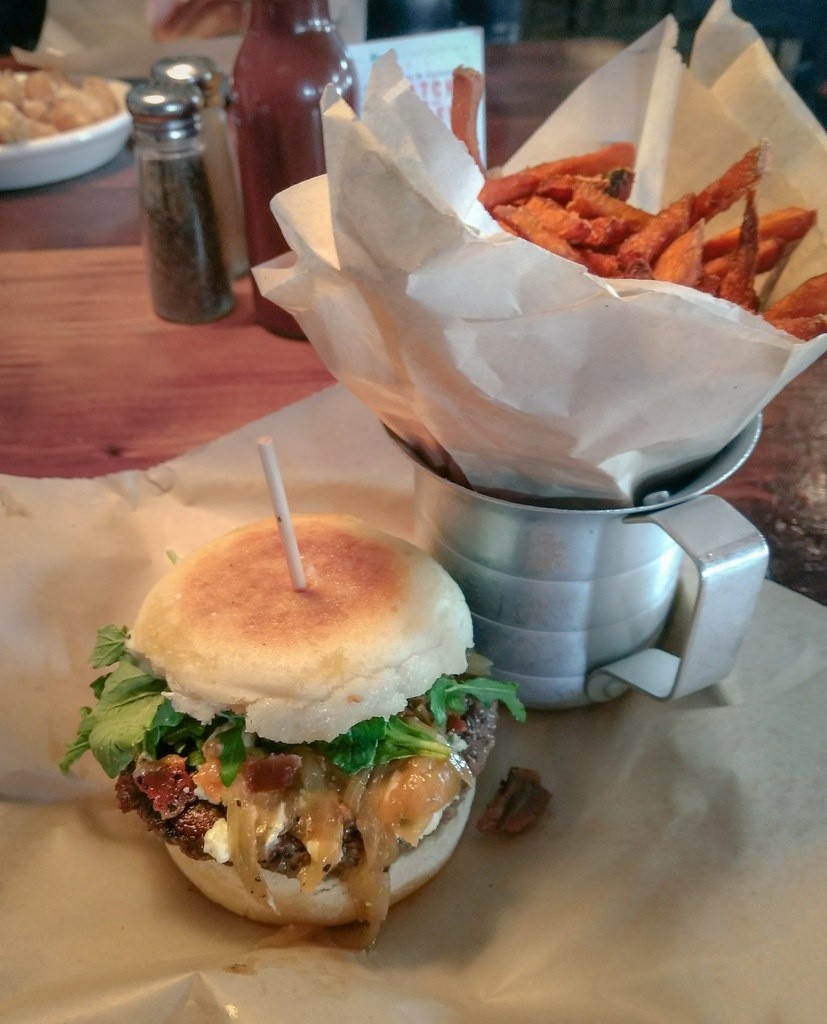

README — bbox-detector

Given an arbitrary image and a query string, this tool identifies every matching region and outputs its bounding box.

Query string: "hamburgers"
[57,512,527,954]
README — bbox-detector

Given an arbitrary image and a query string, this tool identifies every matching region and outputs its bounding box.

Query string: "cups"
[382,417,772,713]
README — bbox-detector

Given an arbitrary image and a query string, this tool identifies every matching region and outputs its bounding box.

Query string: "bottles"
[125,84,234,321]
[233,0,359,340]
[151,55,250,281]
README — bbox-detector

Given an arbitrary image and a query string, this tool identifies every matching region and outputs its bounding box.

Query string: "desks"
[0,41,827,606]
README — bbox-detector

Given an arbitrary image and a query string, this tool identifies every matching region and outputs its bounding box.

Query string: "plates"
[0,73,135,194]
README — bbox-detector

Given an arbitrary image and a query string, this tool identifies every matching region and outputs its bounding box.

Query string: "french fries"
[453,67,827,343]
